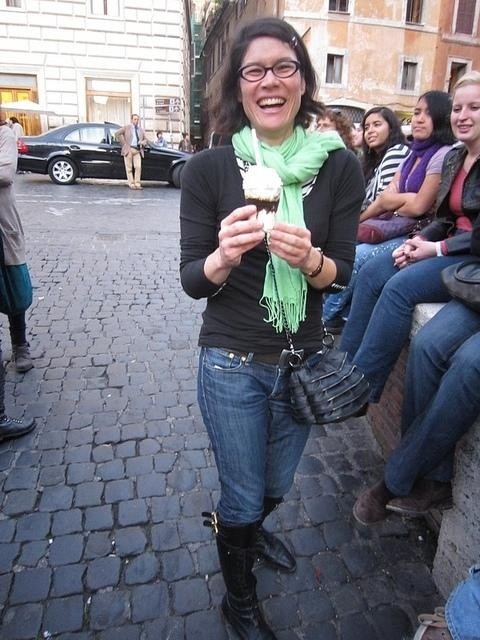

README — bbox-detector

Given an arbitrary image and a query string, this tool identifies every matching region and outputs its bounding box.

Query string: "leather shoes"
[136,186,144,190]
[386,478,454,516]
[12,343,33,372]
[128,184,137,190]
[1,414,37,441]
[353,479,398,526]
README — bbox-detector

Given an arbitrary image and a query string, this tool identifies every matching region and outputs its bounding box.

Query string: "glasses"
[332,108,342,115]
[237,59,302,83]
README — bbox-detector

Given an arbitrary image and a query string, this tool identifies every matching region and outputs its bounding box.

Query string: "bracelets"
[434,241,443,258]
[300,249,324,279]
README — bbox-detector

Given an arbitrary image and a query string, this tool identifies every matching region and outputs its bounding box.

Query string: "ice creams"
[243,166,282,232]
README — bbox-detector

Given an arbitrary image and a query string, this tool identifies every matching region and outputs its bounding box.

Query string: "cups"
[242,182,284,233]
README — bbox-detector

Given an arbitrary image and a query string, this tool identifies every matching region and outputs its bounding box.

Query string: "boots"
[201,509,278,640]
[253,497,298,574]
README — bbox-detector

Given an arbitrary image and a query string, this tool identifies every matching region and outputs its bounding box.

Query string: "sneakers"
[317,314,348,334]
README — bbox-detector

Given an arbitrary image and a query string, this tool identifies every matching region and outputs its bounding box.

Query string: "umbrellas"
[324,96,370,112]
[0,98,56,133]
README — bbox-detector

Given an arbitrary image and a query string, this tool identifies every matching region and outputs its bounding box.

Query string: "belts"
[130,146,140,150]
[221,349,314,369]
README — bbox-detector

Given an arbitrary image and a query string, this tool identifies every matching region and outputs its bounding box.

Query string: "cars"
[10,120,187,185]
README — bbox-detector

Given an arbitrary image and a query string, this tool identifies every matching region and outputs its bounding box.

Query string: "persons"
[115,111,148,188]
[0,123,37,375]
[176,16,365,639]
[335,69,480,420]
[9,115,26,141]
[1,349,41,442]
[153,127,200,158]
[351,250,480,532]
[308,88,458,418]
[410,560,480,639]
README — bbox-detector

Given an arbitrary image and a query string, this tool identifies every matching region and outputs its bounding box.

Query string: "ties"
[135,127,140,151]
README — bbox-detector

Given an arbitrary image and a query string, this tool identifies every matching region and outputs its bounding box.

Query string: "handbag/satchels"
[440,257,480,311]
[289,343,376,426]
[357,208,430,245]
